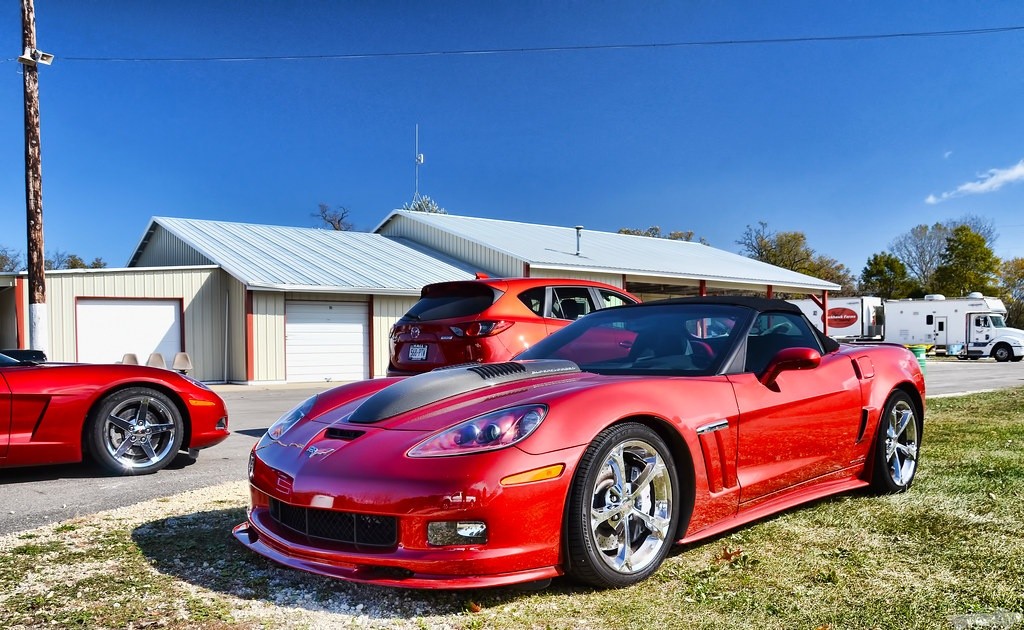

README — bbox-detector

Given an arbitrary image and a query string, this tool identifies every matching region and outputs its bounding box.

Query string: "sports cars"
[230,294,932,589]
[0,348,231,476]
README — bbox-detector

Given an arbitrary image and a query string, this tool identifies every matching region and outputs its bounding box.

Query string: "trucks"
[783,292,1024,363]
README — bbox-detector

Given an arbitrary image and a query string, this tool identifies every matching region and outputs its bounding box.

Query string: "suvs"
[386,273,714,378]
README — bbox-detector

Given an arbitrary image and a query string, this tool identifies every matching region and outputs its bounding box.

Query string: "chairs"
[146,352,166,370]
[121,354,137,365]
[628,326,693,359]
[557,297,579,320]
[172,352,192,375]
